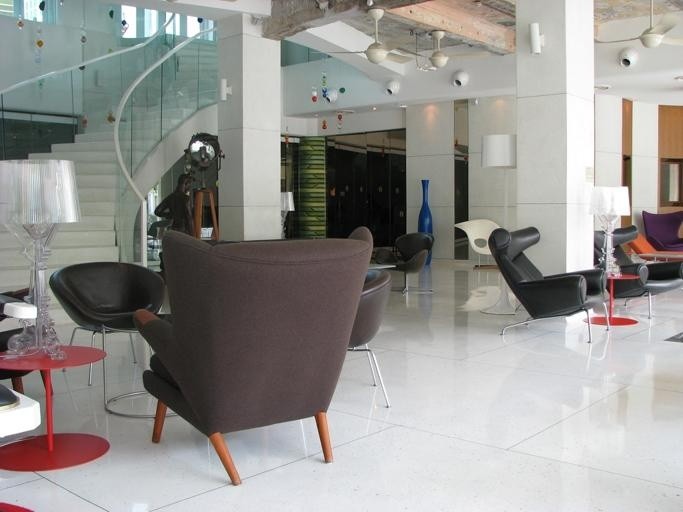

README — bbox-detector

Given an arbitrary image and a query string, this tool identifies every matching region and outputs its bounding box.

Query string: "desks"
[585,274,641,326]
[0,346,110,472]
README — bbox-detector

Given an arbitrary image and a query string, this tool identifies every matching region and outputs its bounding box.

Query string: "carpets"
[663,331,683,342]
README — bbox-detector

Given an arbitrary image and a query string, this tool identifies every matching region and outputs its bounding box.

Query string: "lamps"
[588,186,631,277]
[530,23,545,54]
[0,158,79,362]
[429,52,449,68]
[451,69,470,87]
[324,90,338,102]
[220,78,232,101]
[365,41,388,64]
[639,28,664,49]
[616,47,639,69]
[280,191,295,240]
[385,80,402,97]
[482,134,516,168]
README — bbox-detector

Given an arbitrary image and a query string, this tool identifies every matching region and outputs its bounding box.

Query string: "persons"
[154,174,198,238]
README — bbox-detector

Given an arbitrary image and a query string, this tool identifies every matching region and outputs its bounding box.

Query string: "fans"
[595,0,683,49]
[310,6,414,64]
[397,30,490,68]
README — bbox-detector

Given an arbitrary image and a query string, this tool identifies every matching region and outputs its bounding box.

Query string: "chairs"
[488,227,612,343]
[596,210,683,319]
[454,219,501,270]
[375,233,434,294]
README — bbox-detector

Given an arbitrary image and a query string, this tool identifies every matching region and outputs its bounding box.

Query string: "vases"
[418,180,433,265]
